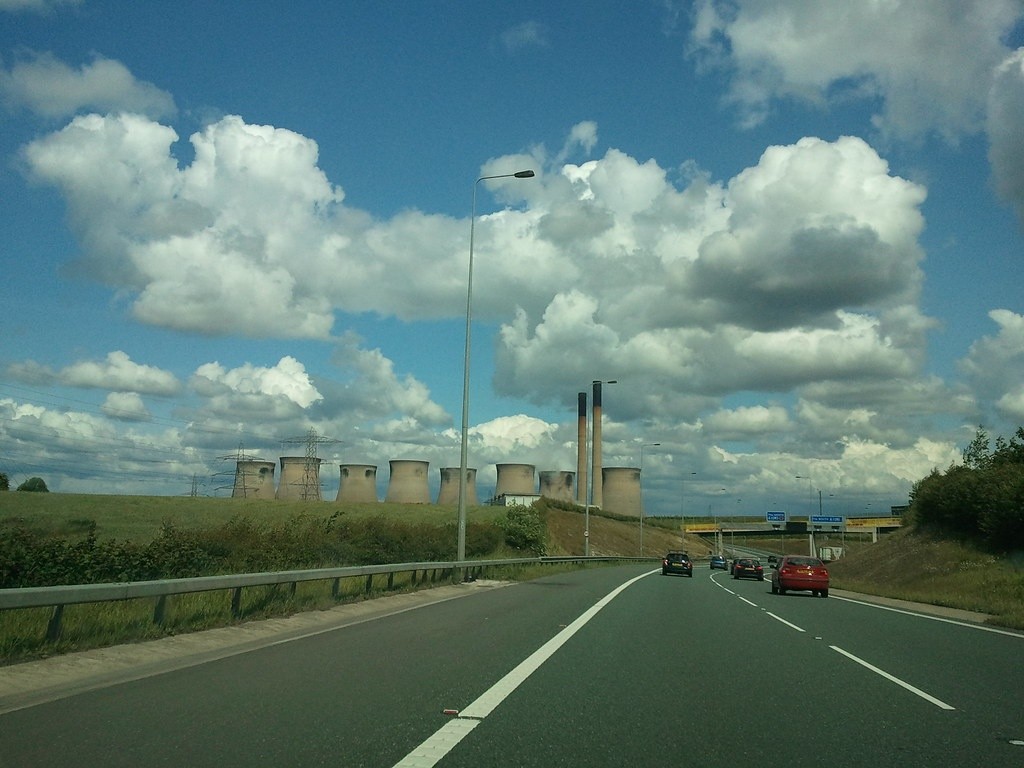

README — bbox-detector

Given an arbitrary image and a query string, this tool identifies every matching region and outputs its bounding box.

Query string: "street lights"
[681,473,696,553]
[796,476,813,556]
[714,489,725,555]
[583,380,617,557]
[457,170,535,561]
[640,443,661,557]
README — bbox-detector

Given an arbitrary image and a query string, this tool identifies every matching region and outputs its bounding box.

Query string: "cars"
[769,555,829,598]
[710,555,728,571]
[768,556,777,563]
[662,553,692,578]
[734,558,763,581]
